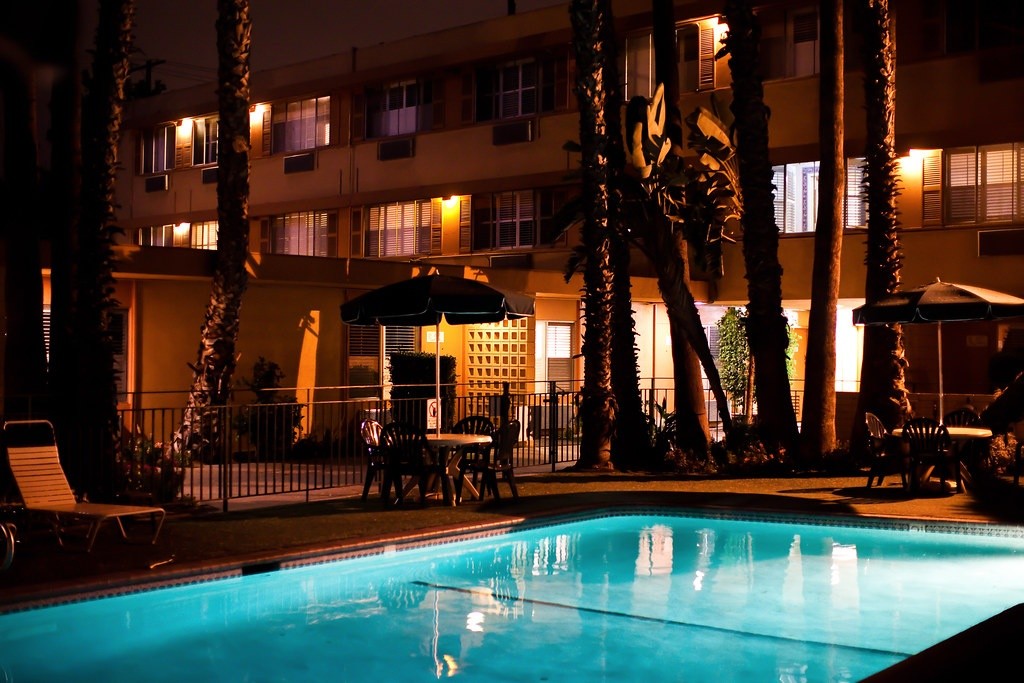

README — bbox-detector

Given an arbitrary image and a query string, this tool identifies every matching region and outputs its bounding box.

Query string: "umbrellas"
[851,270,1024,433]
[339,269,508,436]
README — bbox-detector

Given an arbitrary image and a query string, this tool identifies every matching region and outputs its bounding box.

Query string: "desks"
[893,426,993,490]
[392,434,493,505]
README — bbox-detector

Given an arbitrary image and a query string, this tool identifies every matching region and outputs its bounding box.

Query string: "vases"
[121,437,158,492]
[153,445,191,502]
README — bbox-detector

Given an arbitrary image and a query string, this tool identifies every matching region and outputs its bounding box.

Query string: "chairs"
[864,405,978,493]
[4,420,165,553]
[360,416,520,508]
